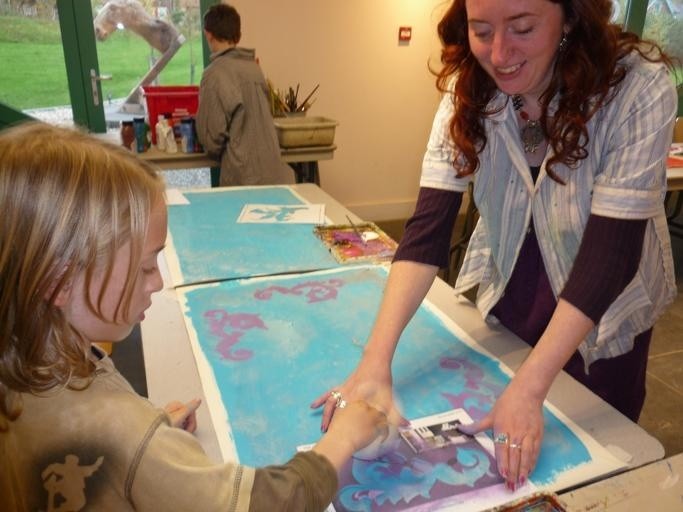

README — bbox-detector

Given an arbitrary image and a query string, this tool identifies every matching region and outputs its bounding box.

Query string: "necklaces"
[512,95,550,154]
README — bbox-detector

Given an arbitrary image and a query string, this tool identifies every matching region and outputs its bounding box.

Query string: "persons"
[193,1,291,185]
[308,1,682,495]
[0,117,390,511]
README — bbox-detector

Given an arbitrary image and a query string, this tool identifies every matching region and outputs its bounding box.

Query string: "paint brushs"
[267,79,320,117]
[346,214,368,246]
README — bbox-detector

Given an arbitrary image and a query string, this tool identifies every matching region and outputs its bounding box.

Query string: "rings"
[330,390,343,400]
[510,445,521,449]
[335,400,347,408]
[493,433,508,444]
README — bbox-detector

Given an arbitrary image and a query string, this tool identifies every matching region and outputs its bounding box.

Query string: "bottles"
[121,115,192,154]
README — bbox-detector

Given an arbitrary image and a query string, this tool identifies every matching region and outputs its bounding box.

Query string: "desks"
[157,182,399,289]
[557,451,683,512]
[140,260,667,512]
